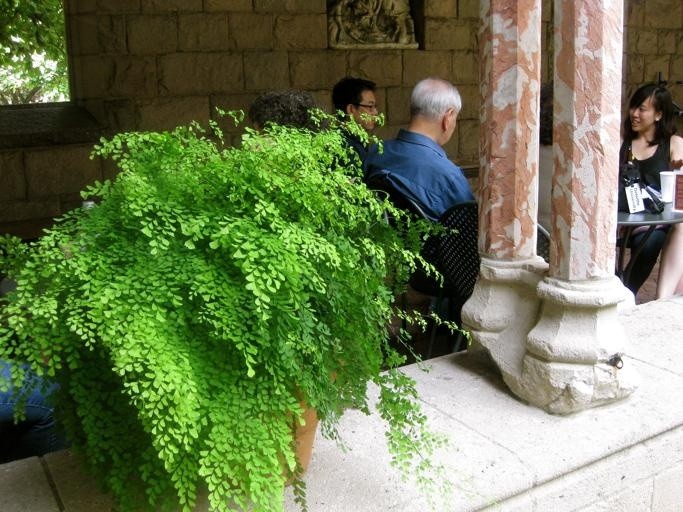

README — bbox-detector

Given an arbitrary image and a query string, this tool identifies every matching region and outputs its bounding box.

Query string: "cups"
[660,171,677,202]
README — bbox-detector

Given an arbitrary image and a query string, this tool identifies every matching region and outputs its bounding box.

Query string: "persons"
[538,81,553,233]
[248,90,315,132]
[617,84,683,296]
[331,77,378,175]
[0,361,62,457]
[364,78,476,321]
[655,222,683,299]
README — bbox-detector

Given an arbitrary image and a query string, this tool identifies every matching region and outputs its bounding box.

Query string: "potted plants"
[0,107,472,512]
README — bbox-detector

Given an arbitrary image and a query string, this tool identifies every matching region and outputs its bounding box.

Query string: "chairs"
[367,180,549,358]
